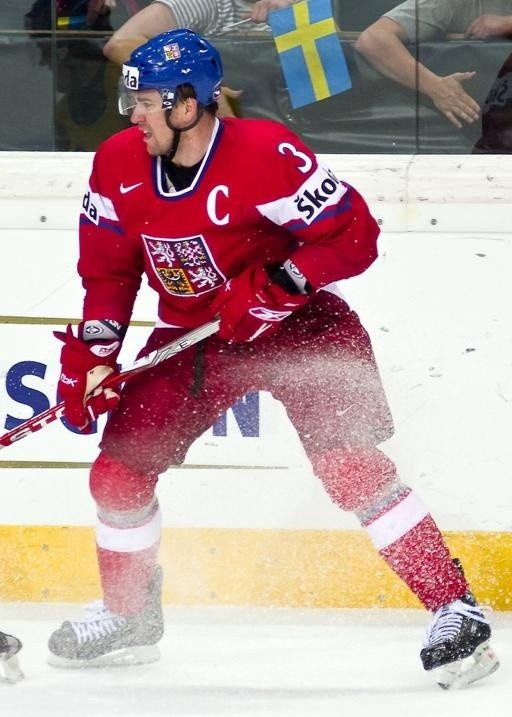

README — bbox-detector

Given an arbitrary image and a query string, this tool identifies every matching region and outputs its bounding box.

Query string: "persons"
[349,0,511,146]
[41,28,490,668]
[105,0,303,126]
[24,0,134,150]
[472,51,510,154]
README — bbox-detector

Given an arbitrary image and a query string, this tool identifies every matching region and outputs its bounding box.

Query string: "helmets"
[122,27,222,110]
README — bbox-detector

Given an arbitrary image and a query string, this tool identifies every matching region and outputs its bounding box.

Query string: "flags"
[266,0,353,112]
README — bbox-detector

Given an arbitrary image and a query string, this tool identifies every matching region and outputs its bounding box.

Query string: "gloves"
[210,263,316,343]
[53,324,121,433]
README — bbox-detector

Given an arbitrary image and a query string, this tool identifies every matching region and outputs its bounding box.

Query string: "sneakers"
[48,565,163,659]
[420,557,491,670]
